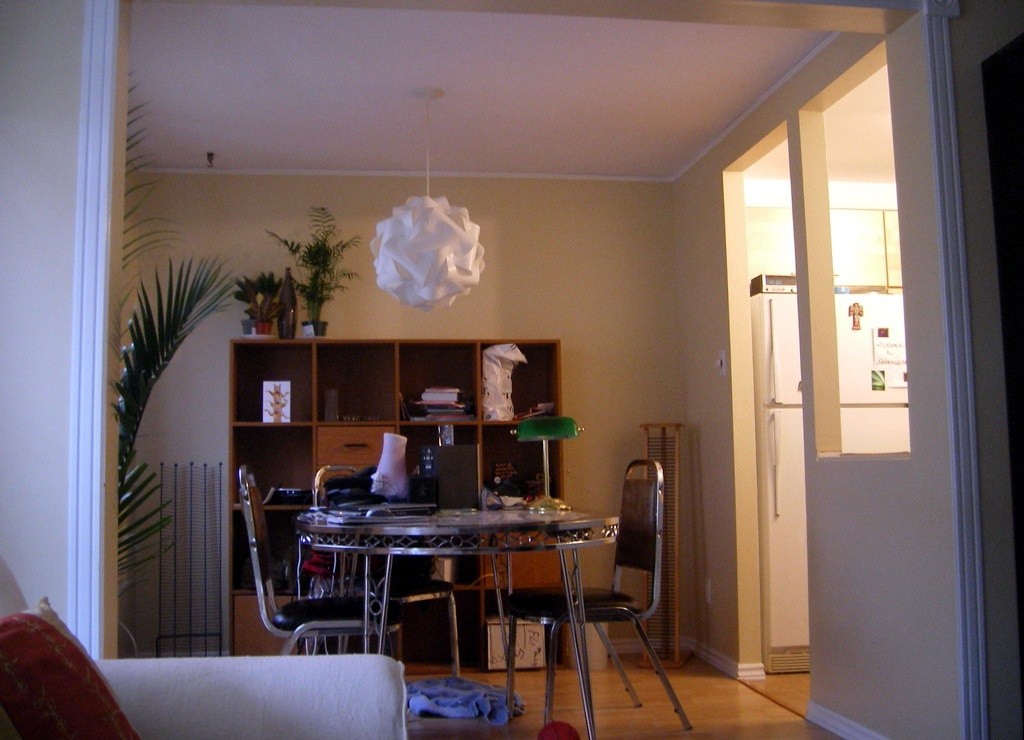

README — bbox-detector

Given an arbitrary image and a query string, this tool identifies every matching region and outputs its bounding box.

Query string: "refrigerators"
[750,293,910,673]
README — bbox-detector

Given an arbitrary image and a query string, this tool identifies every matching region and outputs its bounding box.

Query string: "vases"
[277,266,298,339]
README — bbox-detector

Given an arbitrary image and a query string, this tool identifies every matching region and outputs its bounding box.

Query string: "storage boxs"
[485,617,547,671]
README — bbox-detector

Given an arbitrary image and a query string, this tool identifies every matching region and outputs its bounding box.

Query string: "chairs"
[237,465,403,655]
[312,466,461,678]
[501,459,694,740]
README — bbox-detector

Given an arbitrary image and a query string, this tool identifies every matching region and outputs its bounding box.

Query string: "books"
[409,400,476,421]
[414,386,462,401]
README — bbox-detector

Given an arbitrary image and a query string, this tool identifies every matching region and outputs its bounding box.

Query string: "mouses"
[365,507,395,518]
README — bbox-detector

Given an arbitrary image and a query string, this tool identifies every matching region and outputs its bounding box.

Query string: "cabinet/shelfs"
[883,209,903,294]
[745,204,888,292]
[228,338,572,675]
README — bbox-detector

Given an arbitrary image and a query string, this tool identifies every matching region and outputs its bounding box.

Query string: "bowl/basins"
[278,488,312,505]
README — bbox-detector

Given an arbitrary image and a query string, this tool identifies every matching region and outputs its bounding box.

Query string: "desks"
[296,508,620,740]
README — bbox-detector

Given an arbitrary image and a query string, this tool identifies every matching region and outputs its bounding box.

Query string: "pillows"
[0,595,143,740]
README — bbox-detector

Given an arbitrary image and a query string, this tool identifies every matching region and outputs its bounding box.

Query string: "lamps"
[370,85,486,313]
[510,416,584,512]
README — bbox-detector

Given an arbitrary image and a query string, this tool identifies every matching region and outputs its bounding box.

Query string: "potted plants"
[264,206,363,337]
[232,271,280,335]
[240,308,256,335]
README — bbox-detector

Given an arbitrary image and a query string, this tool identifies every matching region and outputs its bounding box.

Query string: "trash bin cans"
[562,622,608,671]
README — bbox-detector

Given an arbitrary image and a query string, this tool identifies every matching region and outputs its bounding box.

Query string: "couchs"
[0,556,408,740]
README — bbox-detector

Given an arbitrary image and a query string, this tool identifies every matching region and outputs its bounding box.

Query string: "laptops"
[337,504,437,518]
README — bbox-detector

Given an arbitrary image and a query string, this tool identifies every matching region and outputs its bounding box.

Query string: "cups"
[323,388,338,420]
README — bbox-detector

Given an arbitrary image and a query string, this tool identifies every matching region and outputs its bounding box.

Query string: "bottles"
[277,267,296,339]
